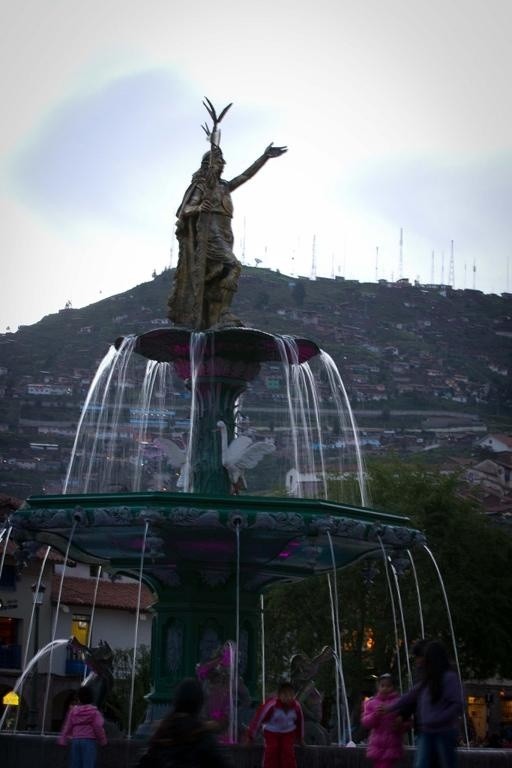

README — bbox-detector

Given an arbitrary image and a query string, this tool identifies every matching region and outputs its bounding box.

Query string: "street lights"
[32,578,47,729]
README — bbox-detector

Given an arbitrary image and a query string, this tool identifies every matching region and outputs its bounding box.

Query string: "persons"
[362,638,464,768]
[243,683,306,768]
[59,687,108,768]
[167,141,288,330]
[140,680,223,768]
